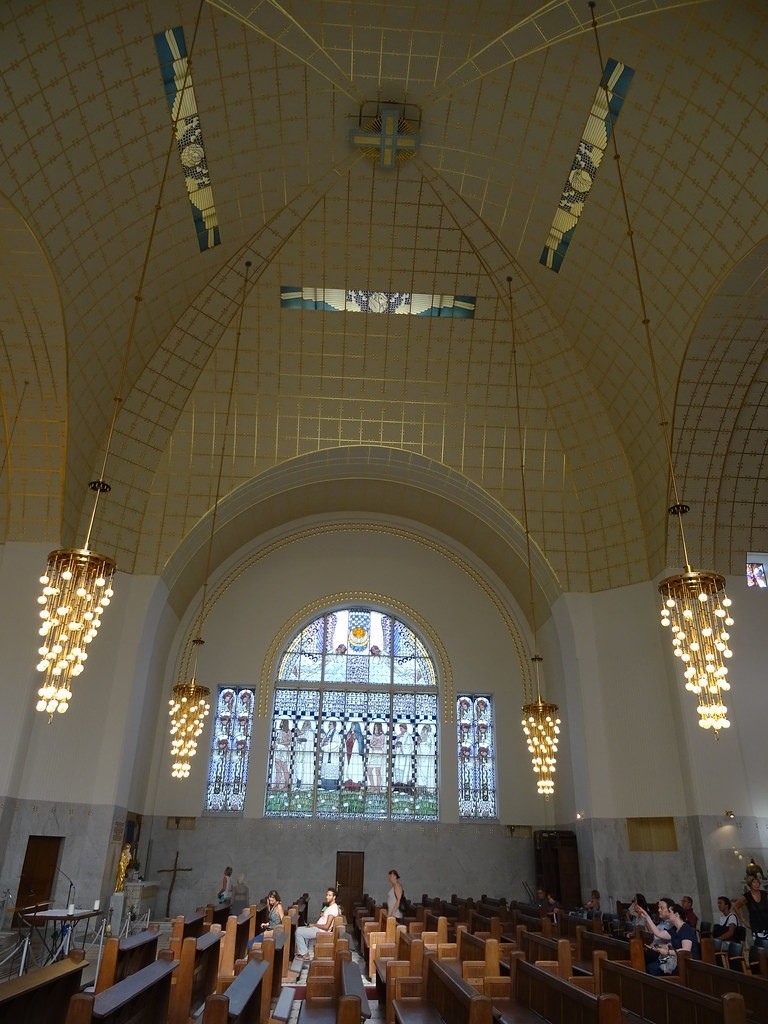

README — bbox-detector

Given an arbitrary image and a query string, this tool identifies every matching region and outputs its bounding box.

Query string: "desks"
[124,880,159,921]
[23,909,105,974]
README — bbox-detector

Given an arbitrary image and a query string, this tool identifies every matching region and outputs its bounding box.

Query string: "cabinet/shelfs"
[533,830,580,908]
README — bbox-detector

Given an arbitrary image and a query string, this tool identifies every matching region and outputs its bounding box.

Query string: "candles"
[92,899,100,912]
[67,903,74,916]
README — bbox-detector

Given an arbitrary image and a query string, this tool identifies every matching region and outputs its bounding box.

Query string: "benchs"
[0,892,768,1024]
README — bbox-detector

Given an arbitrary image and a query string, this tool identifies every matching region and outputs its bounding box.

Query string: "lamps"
[506,275,561,801]
[169,261,253,781]
[587,0,735,742]
[36,0,204,724]
[725,810,735,819]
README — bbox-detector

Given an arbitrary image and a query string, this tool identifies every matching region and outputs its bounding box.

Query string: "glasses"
[632,900,635,903]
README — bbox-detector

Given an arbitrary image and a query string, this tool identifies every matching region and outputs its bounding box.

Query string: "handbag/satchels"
[394,883,407,913]
[712,914,746,942]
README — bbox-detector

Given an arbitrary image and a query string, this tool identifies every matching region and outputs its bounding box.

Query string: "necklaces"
[733,875,768,945]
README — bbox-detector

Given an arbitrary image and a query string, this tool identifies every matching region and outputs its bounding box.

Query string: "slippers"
[295,954,310,961]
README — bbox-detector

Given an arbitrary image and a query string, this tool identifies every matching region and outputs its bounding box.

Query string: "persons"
[115,843,132,892]
[217,866,232,904]
[387,870,403,927]
[537,887,740,977]
[295,887,338,960]
[247,890,284,953]
